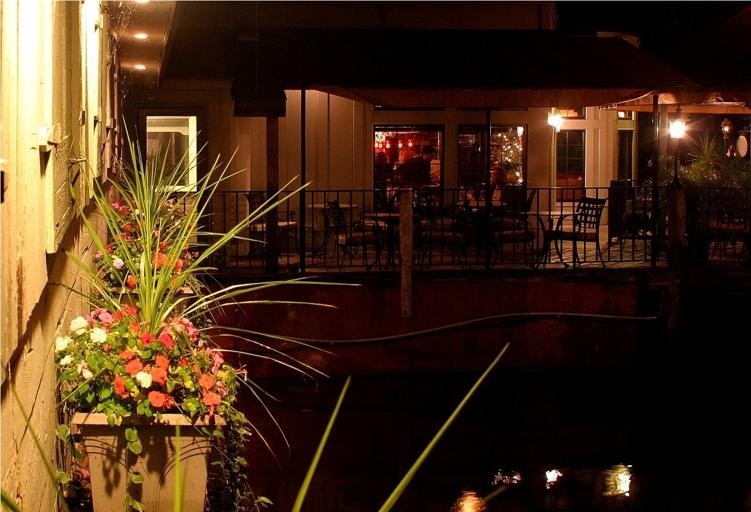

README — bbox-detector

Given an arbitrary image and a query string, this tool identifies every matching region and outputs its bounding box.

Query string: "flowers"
[43,114,363,511]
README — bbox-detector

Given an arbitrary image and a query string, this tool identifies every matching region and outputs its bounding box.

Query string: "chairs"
[243,177,660,272]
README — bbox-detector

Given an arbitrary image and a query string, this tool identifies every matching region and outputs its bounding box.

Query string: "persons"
[483,161,526,200]
[399,144,439,186]
[374,150,397,212]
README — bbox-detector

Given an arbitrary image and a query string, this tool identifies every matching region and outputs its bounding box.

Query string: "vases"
[70,411,229,512]
